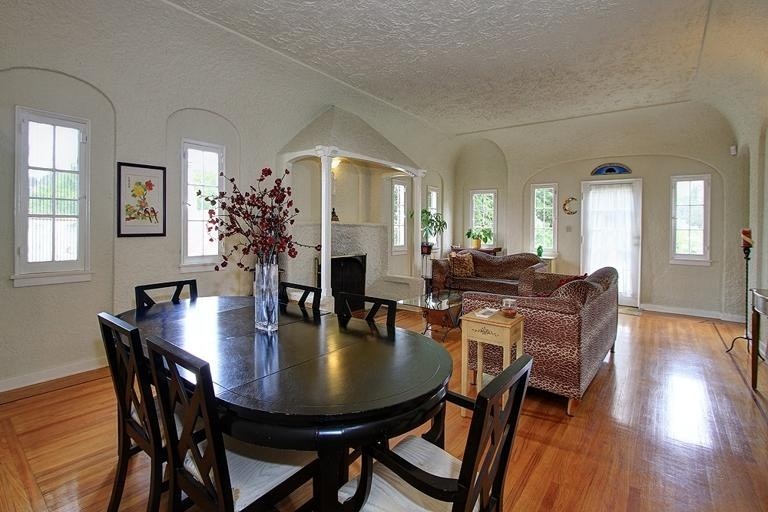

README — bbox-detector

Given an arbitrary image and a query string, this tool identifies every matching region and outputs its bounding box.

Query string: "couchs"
[461,267,621,418]
[432,249,544,292]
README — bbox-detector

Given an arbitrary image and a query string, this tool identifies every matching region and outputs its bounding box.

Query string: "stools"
[459,308,524,420]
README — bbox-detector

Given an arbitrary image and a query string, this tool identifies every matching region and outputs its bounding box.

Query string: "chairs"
[275,280,322,325]
[337,290,396,333]
[133,278,200,308]
[93,311,226,512]
[340,352,535,509]
[143,332,318,512]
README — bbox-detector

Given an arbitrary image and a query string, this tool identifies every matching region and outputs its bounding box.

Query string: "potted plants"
[466,227,491,249]
[410,206,446,255]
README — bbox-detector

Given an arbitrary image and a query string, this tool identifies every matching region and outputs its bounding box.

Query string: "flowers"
[196,167,322,273]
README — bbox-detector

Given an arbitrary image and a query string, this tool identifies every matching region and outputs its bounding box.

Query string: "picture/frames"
[118,161,167,239]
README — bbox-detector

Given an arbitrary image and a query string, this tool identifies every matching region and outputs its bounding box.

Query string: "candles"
[740,225,753,241]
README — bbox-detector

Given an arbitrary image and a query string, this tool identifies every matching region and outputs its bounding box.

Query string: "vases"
[253,264,280,334]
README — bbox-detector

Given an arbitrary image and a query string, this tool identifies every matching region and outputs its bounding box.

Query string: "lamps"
[330,157,344,175]
